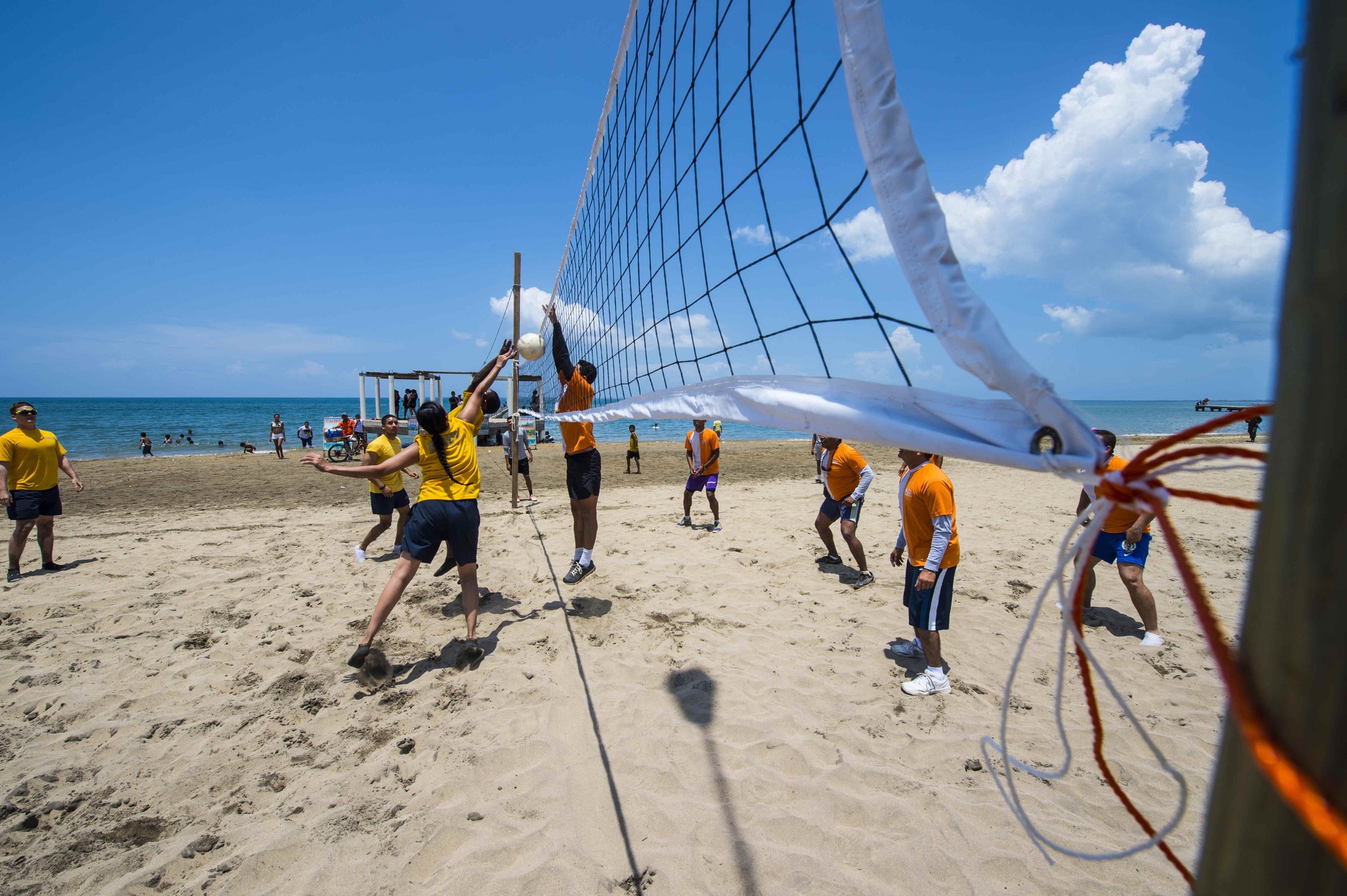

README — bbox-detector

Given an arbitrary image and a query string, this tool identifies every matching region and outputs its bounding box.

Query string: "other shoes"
[517,495,521,502]
[529,494,538,501]
[813,479,821,484]
[1251,440,1255,442]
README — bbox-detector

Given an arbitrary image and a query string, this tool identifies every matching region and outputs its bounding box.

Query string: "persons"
[140,297,1263,694]
[0,402,85,582]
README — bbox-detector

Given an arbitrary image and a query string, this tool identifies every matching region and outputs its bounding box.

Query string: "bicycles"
[328,432,369,463]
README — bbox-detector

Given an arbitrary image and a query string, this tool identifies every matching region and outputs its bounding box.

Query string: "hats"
[342,413,348,417]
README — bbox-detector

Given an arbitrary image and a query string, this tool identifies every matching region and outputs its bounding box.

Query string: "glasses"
[821,466,832,472]
[14,409,37,416]
[483,392,488,404]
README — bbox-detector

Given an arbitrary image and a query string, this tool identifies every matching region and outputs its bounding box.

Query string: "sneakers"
[892,638,927,658]
[562,559,596,585]
[712,521,722,533]
[901,672,951,695]
[815,553,843,565]
[676,517,693,527]
[854,572,876,588]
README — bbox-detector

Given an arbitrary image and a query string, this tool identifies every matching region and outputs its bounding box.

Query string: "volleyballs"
[516,333,546,361]
